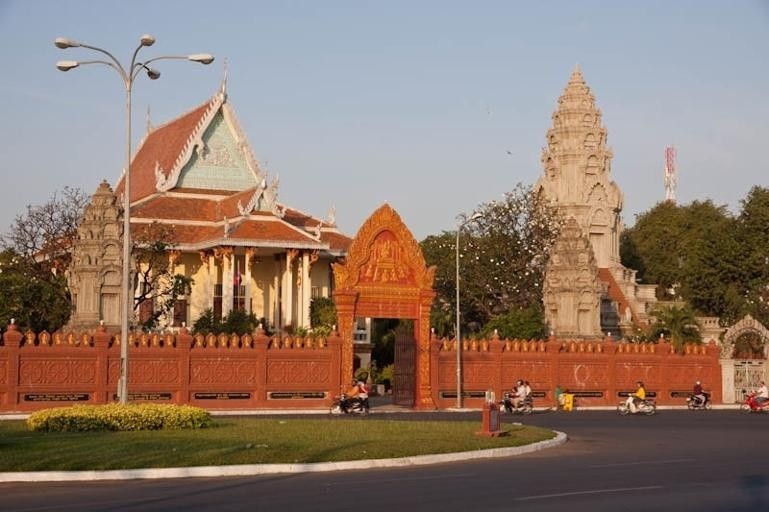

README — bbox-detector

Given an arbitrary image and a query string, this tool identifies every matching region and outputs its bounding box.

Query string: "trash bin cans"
[561,393,575,411]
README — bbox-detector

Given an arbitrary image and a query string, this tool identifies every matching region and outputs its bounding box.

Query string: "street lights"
[456,213,483,408]
[55,35,215,405]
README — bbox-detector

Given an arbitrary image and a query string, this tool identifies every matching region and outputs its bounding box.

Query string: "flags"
[234,268,242,287]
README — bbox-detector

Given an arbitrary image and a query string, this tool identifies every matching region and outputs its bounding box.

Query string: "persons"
[508,380,533,414]
[632,381,645,411]
[553,385,561,411]
[752,381,769,410]
[345,380,369,413]
[694,381,706,407]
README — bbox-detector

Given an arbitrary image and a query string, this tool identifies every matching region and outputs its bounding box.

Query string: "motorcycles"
[330,395,368,417]
[498,393,532,415]
[686,392,712,410]
[740,391,769,413]
[617,394,656,416]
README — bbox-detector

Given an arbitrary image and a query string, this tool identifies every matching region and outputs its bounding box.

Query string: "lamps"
[249,247,264,265]
